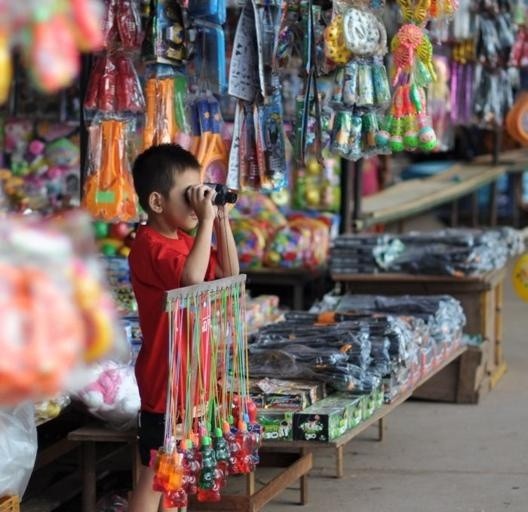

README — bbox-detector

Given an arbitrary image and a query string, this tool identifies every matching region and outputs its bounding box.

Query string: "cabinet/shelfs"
[355,147,528,235]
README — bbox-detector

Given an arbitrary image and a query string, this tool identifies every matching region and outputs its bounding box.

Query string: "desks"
[336,271,506,403]
[68,423,140,512]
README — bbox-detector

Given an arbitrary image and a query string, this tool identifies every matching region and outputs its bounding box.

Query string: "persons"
[126,143,242,511]
